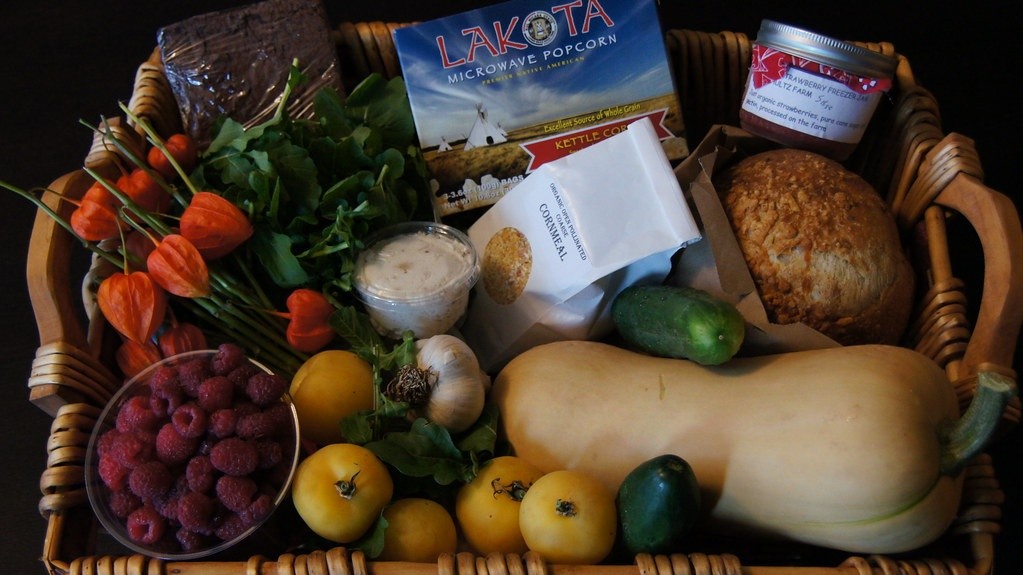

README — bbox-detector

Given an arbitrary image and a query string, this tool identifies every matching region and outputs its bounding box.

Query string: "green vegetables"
[196,47,425,301]
[329,308,495,558]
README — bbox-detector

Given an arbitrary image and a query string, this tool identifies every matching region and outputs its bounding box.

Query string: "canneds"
[740,19,903,164]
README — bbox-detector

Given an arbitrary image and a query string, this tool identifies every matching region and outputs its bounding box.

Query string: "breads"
[716,145,913,347]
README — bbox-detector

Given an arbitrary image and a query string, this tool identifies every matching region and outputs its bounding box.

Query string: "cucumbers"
[612,454,704,566]
[608,284,747,366]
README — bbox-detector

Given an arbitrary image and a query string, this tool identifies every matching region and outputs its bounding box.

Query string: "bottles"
[738,17,900,163]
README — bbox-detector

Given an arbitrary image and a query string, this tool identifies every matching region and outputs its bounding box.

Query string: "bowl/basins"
[346,220,481,341]
[82,348,302,562]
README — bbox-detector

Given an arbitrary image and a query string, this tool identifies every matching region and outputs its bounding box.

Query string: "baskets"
[26,11,1023,573]
